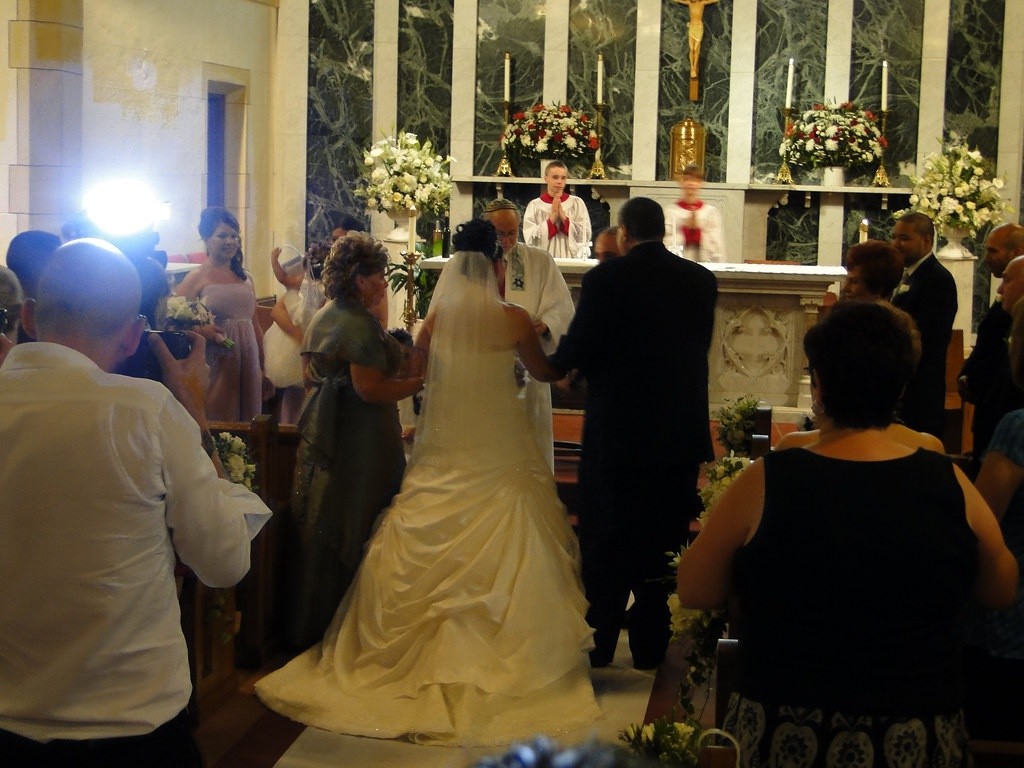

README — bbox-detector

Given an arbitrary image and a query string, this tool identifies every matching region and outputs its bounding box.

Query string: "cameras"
[112,328,192,382]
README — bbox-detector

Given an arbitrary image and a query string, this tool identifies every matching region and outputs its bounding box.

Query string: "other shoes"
[240,676,263,695]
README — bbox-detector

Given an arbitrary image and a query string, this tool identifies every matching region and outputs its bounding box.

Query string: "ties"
[897,272,908,293]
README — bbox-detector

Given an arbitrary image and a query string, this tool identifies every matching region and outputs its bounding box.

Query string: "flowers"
[888,128,1014,240]
[696,454,759,530]
[779,101,889,172]
[212,432,256,494]
[350,131,451,216]
[714,388,765,454]
[661,541,731,721]
[165,294,236,351]
[501,102,601,159]
[615,715,710,768]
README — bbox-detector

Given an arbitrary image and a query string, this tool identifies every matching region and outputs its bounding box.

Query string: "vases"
[384,207,423,241]
[935,222,974,260]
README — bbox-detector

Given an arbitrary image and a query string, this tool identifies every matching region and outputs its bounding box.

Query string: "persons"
[521,162,591,261]
[664,168,728,264]
[0,198,1024,767]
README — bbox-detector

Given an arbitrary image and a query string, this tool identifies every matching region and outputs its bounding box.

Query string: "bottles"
[442,216,451,258]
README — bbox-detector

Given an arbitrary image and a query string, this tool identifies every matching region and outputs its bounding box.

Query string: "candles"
[881,60,888,111]
[785,57,795,108]
[597,54,605,104]
[504,53,511,102]
